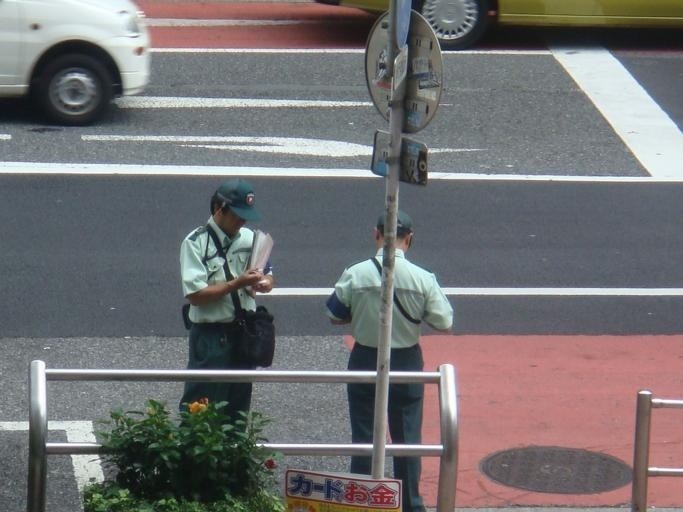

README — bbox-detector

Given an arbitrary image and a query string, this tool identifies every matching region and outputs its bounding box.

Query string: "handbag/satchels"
[233,306,276,368]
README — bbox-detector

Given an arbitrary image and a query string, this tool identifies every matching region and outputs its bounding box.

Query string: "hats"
[216,180,264,221]
[378,209,414,232]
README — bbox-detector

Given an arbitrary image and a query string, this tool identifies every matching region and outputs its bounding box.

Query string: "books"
[243,229,273,299]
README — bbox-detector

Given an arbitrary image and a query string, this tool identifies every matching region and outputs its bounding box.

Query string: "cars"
[0,1,152,126]
[314,0,683,51]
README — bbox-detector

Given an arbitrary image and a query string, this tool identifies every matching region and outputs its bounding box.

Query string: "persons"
[325,209,454,511]
[176,177,274,435]
